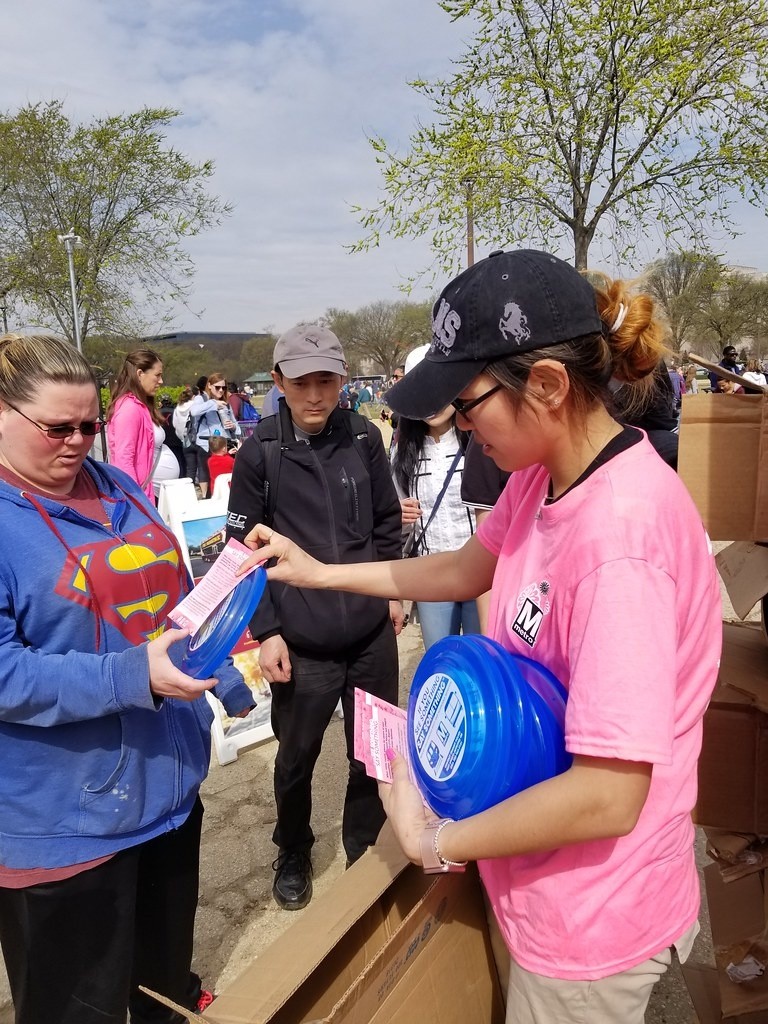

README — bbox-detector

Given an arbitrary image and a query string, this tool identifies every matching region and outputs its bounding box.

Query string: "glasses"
[212,384,228,391]
[1,397,107,438]
[730,352,738,356]
[450,384,504,423]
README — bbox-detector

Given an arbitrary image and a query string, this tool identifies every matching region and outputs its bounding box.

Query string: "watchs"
[419,818,465,874]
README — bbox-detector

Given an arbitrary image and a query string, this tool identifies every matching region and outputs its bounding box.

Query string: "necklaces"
[534,418,614,520]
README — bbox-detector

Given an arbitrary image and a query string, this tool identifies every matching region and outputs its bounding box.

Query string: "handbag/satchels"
[241,398,261,420]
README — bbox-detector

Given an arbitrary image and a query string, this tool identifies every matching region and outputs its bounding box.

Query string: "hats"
[237,386,244,390]
[404,342,431,375]
[273,324,347,379]
[159,393,172,400]
[384,249,602,420]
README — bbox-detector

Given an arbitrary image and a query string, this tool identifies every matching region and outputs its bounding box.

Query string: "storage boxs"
[138,846,505,1024]
[692,621,768,836]
[681,853,768,1024]
[678,353,768,621]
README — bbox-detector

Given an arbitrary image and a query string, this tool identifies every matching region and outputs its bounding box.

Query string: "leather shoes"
[272,846,313,910]
[346,860,352,870]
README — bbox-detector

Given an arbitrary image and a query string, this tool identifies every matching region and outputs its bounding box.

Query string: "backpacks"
[185,394,230,445]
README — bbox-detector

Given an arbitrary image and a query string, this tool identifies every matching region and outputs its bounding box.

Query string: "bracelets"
[434,819,468,866]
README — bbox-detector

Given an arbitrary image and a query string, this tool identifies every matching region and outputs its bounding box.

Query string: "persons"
[225,323,406,911]
[0,344,768,1024]
[0,334,257,1024]
[236,249,725,1024]
